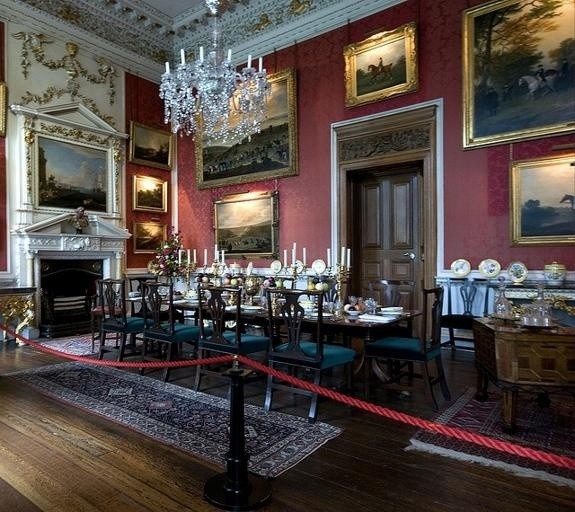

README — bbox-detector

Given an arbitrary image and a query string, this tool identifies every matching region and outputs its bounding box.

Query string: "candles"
[214,245,217,265]
[293,241,297,267]
[228,49,232,63]
[327,249,331,268]
[217,251,219,266]
[248,55,252,68]
[200,48,204,62]
[188,249,190,266]
[179,244,183,264]
[194,250,197,266]
[260,58,263,76]
[165,62,170,75]
[182,50,185,65]
[283,250,289,270]
[341,247,345,270]
[346,248,352,272]
[222,251,224,267]
[302,249,306,268]
[204,249,208,267]
[263,69,267,73]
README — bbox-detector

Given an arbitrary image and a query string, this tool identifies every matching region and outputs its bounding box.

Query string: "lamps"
[159,0,274,147]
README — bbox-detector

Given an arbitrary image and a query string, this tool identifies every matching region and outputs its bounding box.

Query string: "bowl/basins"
[133,292,142,297]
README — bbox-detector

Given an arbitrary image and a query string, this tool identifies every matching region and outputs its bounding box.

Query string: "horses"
[367,63,393,85]
[518,72,558,101]
[559,194,575,204]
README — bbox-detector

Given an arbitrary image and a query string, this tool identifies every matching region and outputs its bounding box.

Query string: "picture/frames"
[25,114,124,216]
[130,124,174,169]
[342,21,418,108]
[133,175,168,213]
[464,1,575,148]
[133,219,170,254]
[510,153,575,244]
[214,192,276,262]
[196,67,297,189]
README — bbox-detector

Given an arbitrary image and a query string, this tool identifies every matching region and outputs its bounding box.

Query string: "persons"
[535,64,547,83]
[377,57,383,76]
[502,84,513,101]
[485,87,498,115]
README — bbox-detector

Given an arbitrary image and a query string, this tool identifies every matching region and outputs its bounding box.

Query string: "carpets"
[3,358,345,477]
[36,322,144,358]
[411,374,570,482]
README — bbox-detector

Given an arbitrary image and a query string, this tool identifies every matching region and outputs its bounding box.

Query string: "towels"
[359,313,390,321]
[222,304,239,310]
[167,300,187,304]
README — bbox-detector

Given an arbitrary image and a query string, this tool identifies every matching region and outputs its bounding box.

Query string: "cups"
[332,310,343,321]
[518,304,551,326]
[128,292,135,299]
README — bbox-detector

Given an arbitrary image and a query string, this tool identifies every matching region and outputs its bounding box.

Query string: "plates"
[247,262,254,276]
[167,300,190,305]
[312,259,326,274]
[242,305,264,311]
[279,312,308,317]
[449,258,471,276]
[507,261,527,283]
[191,300,207,305]
[346,310,363,316]
[310,313,334,319]
[291,260,304,275]
[478,259,500,278]
[271,260,282,274]
[130,297,149,301]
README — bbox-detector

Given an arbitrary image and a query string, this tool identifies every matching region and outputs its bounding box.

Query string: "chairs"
[123,273,183,347]
[441,278,491,352]
[139,281,210,378]
[97,279,155,364]
[368,287,451,403]
[260,287,357,419]
[194,286,272,391]
